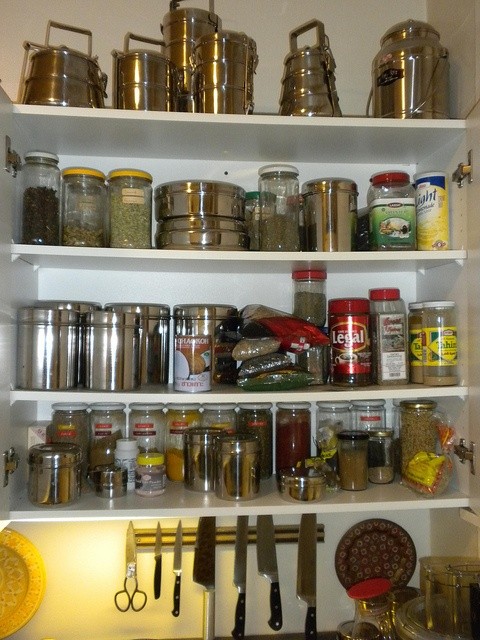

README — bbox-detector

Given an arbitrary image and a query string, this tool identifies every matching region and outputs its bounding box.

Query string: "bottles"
[328,297,372,387]
[129,401,166,452]
[203,404,236,436]
[107,168,153,249]
[59,166,107,248]
[20,150,61,245]
[316,401,352,436]
[112,439,139,498]
[366,170,416,250]
[339,426,369,491]
[407,297,457,384]
[245,164,301,252]
[160,403,202,449]
[51,402,89,449]
[413,171,450,250]
[290,269,327,328]
[349,399,388,430]
[236,402,273,480]
[399,397,439,477]
[369,286,410,384]
[276,401,312,478]
[370,426,395,485]
[133,448,168,498]
[349,585,396,640]
[88,402,127,480]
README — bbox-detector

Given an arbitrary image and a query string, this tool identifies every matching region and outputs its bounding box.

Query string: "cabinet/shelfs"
[1,2,480,635]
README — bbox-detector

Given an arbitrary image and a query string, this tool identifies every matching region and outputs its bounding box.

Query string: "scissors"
[112,519,148,616]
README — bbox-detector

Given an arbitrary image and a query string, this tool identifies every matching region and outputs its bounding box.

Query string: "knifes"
[170,519,185,617]
[230,515,249,640]
[154,522,164,600]
[256,515,283,631]
[193,516,216,640]
[295,513,327,640]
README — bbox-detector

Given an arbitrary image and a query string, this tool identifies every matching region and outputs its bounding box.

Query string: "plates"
[1,526,47,640]
[334,518,417,595]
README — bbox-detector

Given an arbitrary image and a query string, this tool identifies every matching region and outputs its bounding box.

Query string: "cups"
[419,553,478,639]
